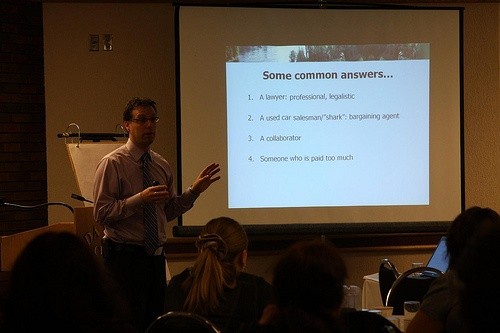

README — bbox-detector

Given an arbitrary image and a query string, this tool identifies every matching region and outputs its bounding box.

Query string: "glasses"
[131,117,159,124]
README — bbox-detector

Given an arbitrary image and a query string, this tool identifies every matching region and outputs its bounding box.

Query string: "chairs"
[378,259,444,315]
[143,311,221,333]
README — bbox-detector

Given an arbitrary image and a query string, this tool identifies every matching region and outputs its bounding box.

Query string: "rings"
[154,192,157,196]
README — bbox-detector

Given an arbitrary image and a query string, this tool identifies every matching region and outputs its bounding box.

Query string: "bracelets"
[190,185,199,194]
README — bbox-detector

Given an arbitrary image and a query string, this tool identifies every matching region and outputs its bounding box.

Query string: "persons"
[160,217,272,333]
[406,206,500,333]
[94,95,220,333]
[0,231,139,333]
[238,239,368,333]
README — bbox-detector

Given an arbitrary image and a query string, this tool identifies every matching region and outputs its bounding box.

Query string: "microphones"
[0,200,75,215]
[71,193,94,204]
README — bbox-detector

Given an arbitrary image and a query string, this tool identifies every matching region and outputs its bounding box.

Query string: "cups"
[374,307,393,316]
[412,263,425,277]
[404,301,420,320]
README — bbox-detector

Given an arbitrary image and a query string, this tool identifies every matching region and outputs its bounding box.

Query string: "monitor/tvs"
[419,235,451,277]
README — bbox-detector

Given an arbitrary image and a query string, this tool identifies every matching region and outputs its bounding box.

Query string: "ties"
[141,153,159,258]
